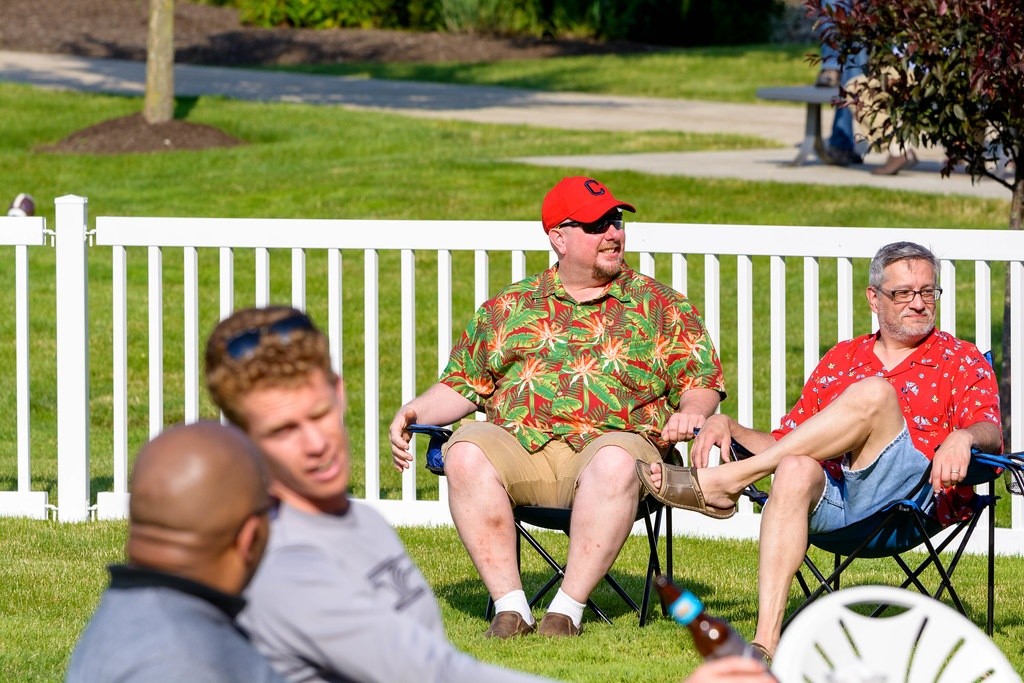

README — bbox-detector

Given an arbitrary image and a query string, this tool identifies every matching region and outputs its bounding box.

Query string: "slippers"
[750,641,772,669]
[635,459,736,519]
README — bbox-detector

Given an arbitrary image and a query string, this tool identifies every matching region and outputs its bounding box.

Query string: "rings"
[951,471,960,474]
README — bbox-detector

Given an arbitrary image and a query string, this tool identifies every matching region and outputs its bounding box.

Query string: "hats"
[542,176,636,234]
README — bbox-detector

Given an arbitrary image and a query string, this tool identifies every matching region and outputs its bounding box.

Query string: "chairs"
[730,349,1023,639]
[407,408,687,630]
[766,586,1024,683]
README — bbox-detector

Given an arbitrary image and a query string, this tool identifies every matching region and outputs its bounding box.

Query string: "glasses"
[232,492,283,549]
[872,283,944,302]
[556,212,622,234]
[212,315,318,373]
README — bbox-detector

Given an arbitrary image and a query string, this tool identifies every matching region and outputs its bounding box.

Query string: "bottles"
[651,574,780,683]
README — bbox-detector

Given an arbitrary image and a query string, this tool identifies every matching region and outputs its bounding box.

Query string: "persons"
[816,0,918,175]
[206,305,778,683]
[65,421,287,683]
[388,178,728,639]
[635,242,1004,670]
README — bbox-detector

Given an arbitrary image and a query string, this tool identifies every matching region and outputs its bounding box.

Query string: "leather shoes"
[485,610,537,640]
[537,613,583,638]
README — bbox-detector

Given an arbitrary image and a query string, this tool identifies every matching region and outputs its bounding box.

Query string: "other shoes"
[815,69,838,85]
[873,149,919,175]
